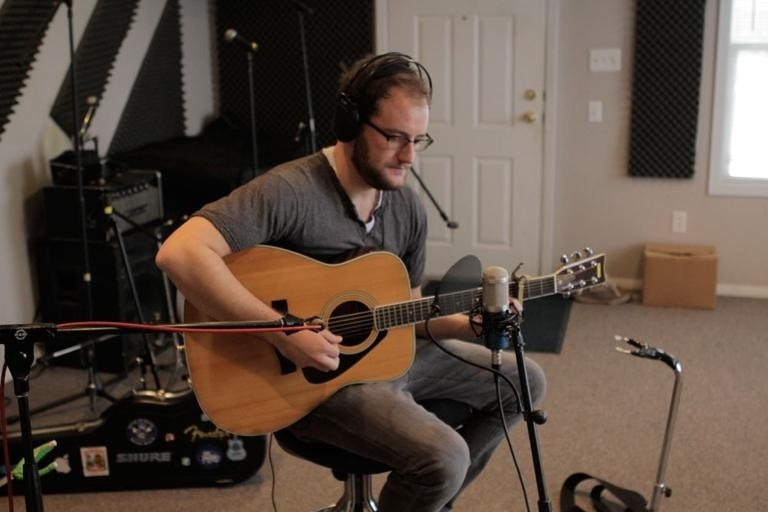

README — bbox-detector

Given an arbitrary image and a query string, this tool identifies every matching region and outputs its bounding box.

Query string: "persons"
[153,52,548,512]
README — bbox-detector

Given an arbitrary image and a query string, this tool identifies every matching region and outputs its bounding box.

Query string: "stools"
[273,400,475,512]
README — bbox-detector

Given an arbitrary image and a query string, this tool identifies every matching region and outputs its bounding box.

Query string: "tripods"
[6,0,118,426]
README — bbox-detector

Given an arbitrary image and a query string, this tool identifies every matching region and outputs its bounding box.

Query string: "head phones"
[333,52,432,144]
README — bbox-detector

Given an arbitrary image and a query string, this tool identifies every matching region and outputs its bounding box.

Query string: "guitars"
[184,244,609,436]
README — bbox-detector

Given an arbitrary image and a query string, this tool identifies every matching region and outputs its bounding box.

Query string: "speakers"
[46,225,167,375]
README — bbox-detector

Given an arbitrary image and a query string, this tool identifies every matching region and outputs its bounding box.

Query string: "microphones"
[224,29,258,54]
[482,265,510,349]
[305,317,325,334]
[410,166,459,228]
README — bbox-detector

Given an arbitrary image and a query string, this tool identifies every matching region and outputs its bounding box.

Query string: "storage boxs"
[640,242,719,311]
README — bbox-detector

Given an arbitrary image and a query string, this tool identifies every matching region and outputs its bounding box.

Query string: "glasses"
[366,120,433,152]
